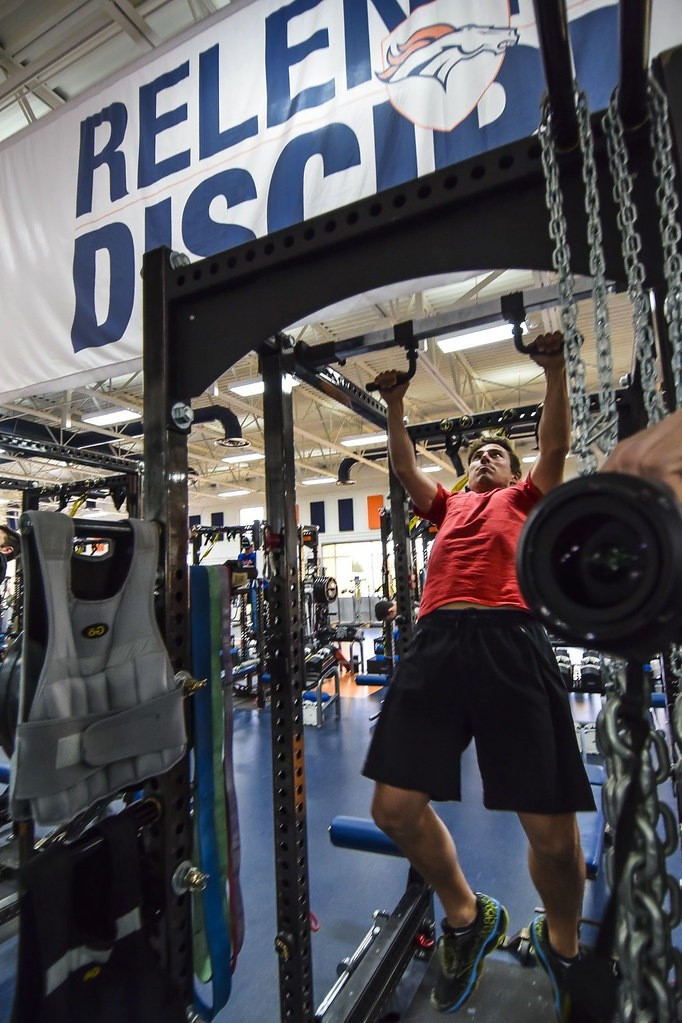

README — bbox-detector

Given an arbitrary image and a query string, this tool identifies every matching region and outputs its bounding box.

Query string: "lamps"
[81,405,142,427]
[229,374,300,397]
[434,316,528,354]
[218,490,250,497]
[302,476,336,485]
[420,464,442,473]
[341,430,388,447]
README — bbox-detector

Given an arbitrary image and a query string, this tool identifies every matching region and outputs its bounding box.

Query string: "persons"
[0,525,22,586]
[238,545,258,579]
[360,330,598,1023]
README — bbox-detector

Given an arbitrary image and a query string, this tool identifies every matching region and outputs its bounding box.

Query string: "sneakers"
[528,915,586,1023]
[430,892,510,1014]
